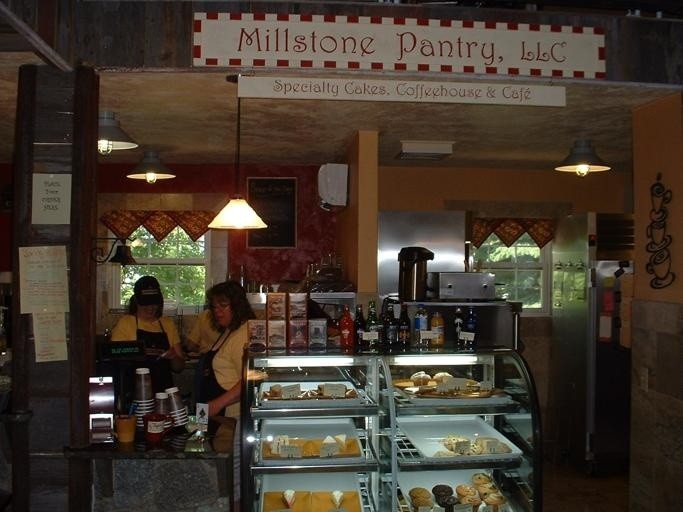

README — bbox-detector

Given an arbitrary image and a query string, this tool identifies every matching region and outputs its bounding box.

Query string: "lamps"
[96,108,137,156]
[207,74,268,231]
[546,133,612,177]
[124,150,177,183]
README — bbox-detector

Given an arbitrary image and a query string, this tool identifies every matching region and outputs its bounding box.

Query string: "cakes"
[411,370,493,394]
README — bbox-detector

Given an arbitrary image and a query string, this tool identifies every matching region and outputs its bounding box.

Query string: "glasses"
[209,303,231,312]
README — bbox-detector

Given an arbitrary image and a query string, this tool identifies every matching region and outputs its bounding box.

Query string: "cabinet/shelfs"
[238,345,541,511]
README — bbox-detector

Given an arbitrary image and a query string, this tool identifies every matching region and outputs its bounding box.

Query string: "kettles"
[399,245,435,300]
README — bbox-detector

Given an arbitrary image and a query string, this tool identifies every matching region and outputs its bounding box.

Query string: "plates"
[648,206,669,222]
[649,271,676,290]
[645,236,672,249]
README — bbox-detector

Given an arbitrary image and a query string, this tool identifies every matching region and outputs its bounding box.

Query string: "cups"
[646,250,670,279]
[651,183,670,212]
[115,366,190,443]
[647,222,668,244]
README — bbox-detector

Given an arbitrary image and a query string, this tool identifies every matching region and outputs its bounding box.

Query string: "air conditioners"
[315,158,349,214]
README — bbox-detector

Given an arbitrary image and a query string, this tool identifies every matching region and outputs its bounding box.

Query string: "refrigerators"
[551,211,632,475]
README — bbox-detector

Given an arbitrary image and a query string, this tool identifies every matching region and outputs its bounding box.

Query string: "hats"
[133,275,162,307]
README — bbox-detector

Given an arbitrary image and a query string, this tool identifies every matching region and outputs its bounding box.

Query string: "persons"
[193,280,257,417]
[108,275,186,413]
[181,307,266,401]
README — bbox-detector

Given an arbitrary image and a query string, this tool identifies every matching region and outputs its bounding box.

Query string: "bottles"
[337,301,480,351]
[237,265,267,293]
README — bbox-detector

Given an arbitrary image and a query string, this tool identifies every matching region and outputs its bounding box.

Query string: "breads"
[282,490,296,507]
[263,383,355,397]
[408,473,507,511]
[330,490,344,508]
[435,435,511,457]
[271,434,304,458]
[321,434,347,457]
